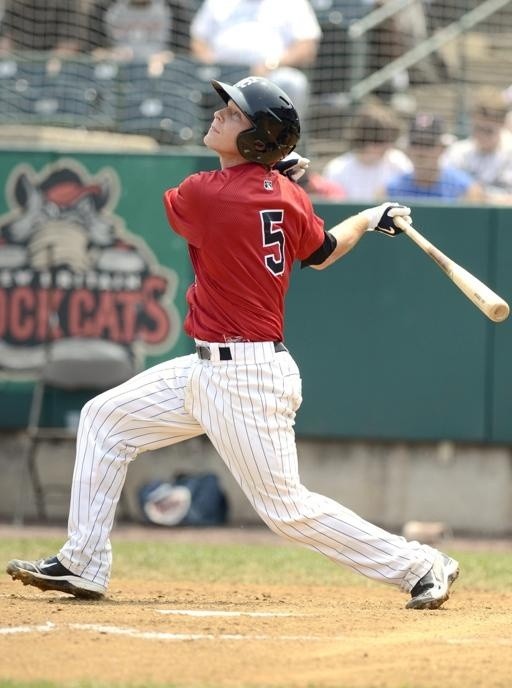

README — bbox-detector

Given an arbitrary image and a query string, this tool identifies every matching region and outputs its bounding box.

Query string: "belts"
[196,341,285,360]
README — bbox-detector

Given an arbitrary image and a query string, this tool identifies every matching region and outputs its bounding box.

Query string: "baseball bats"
[393,216,510,326]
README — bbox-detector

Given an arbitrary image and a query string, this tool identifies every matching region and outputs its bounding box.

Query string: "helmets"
[210,76,300,166]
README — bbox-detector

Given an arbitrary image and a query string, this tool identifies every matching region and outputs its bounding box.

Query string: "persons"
[102,0,173,56]
[378,110,485,201]
[324,111,413,201]
[440,86,511,202]
[4,72,461,610]
[189,0,324,71]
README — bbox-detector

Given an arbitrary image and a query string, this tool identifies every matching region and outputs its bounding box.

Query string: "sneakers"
[406,552,460,609]
[7,557,107,600]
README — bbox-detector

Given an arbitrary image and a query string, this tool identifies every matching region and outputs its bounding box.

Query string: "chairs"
[1,338,139,522]
[0,47,253,146]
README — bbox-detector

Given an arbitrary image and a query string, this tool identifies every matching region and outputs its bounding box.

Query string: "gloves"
[273,151,310,183]
[359,202,412,238]
